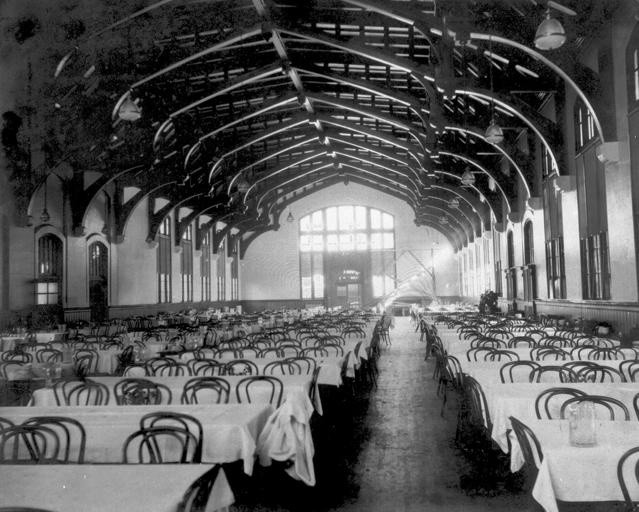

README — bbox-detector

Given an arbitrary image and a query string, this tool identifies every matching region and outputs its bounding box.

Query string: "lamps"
[286,203,294,222]
[39,153,51,222]
[447,40,476,210]
[119,90,141,122]
[532,0,564,55]
[483,26,505,146]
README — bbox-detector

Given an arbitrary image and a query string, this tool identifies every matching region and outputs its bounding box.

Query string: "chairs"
[3,310,390,353]
[455,377,639,511]
[1,351,377,400]
[430,343,639,415]
[1,366,323,512]
[411,303,639,361]
